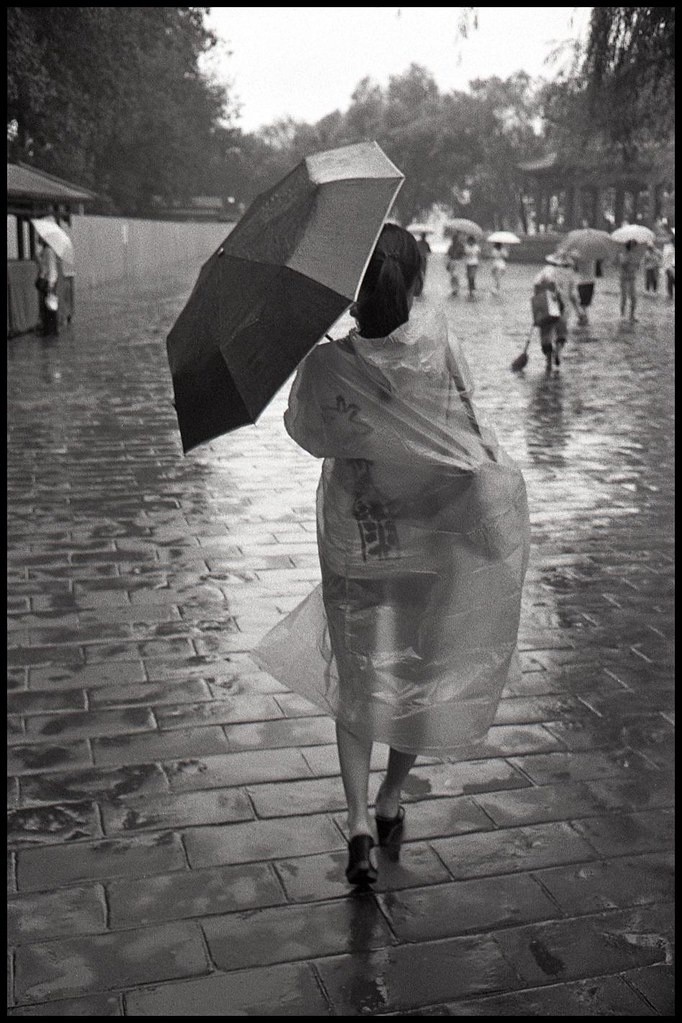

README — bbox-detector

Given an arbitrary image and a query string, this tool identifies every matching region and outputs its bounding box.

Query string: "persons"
[415,233,432,281]
[284,221,470,882]
[463,236,481,299]
[642,242,663,292]
[490,243,508,293]
[662,235,676,297]
[531,250,588,372]
[614,240,643,323]
[574,256,596,328]
[31,235,64,337]
[445,235,466,296]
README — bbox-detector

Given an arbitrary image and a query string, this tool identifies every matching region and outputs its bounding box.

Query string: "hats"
[546,249,574,265]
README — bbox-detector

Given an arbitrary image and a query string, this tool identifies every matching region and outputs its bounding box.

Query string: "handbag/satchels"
[530,291,560,326]
[423,440,526,560]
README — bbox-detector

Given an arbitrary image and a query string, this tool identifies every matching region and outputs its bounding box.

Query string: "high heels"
[375,804,406,847]
[345,836,378,887]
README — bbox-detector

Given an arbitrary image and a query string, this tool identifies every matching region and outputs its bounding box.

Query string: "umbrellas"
[487,230,520,244]
[443,218,482,239]
[165,139,407,459]
[610,224,656,244]
[406,223,435,235]
[32,216,75,264]
[564,226,615,259]
